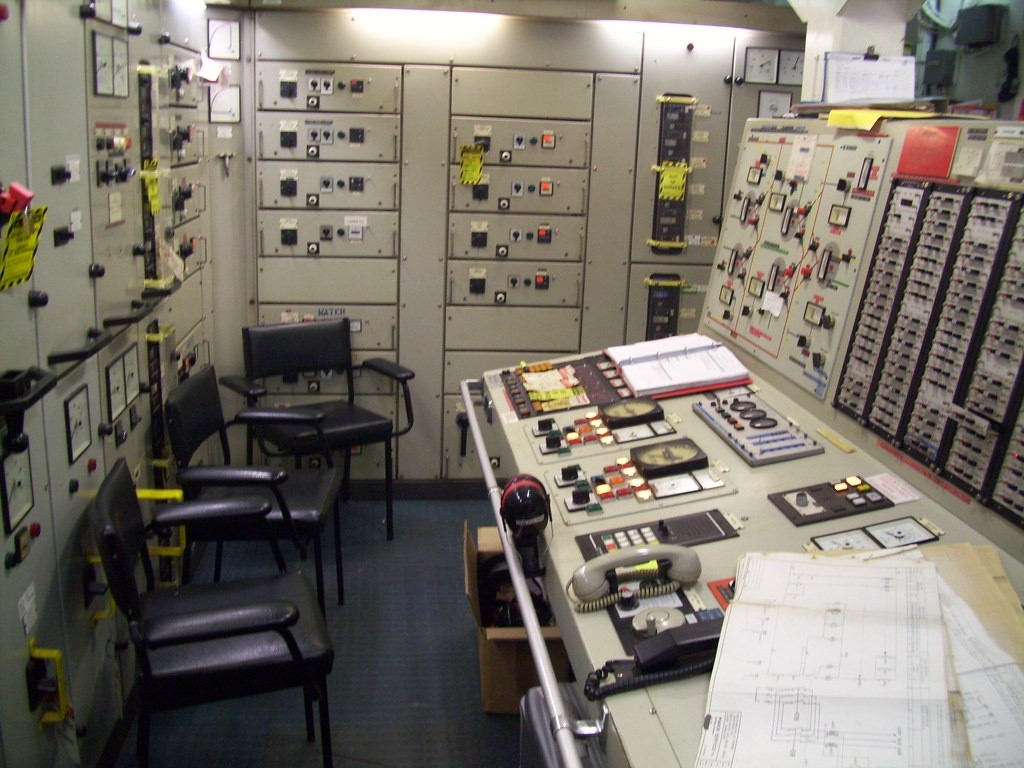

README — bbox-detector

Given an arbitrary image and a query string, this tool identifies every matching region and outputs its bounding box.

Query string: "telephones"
[629,615,726,675]
[564,543,703,615]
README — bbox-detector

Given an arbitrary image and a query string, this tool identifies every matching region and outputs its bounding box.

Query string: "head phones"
[499,473,549,576]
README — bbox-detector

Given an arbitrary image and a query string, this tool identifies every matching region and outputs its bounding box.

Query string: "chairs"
[218,317,415,560]
[91,457,333,768]
[164,365,344,621]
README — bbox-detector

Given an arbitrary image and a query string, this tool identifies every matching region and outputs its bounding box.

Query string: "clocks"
[778,48,805,86]
[744,47,780,85]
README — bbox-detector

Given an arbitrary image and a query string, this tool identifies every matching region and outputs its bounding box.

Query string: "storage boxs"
[520,681,603,768]
[463,519,572,714]
